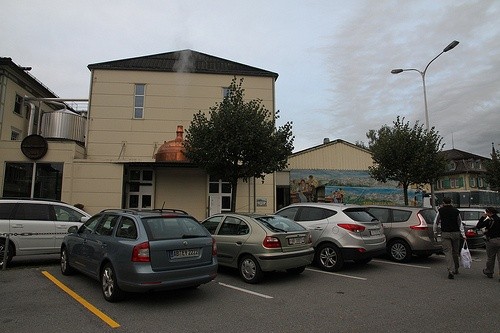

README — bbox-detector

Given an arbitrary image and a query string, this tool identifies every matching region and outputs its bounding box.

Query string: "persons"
[432,196,467,279]
[475,207,500,281]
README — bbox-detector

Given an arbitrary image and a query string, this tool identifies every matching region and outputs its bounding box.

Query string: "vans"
[0,200,131,267]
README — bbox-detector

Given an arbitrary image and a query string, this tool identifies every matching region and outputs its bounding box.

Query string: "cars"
[201,213,315,284]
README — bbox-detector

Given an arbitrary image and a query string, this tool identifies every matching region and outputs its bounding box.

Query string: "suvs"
[60,201,218,302]
[274,203,386,271]
[368,204,488,263]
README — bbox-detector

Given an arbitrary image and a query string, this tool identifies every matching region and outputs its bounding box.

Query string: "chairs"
[110,218,123,227]
[226,219,237,229]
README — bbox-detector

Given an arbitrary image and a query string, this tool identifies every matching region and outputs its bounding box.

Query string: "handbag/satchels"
[460,239,472,269]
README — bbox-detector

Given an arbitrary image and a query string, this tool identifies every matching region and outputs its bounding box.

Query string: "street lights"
[391,41,459,207]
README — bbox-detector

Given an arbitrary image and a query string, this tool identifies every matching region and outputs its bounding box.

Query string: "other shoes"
[483,268,493,278]
[453,268,458,273]
[448,271,454,279]
